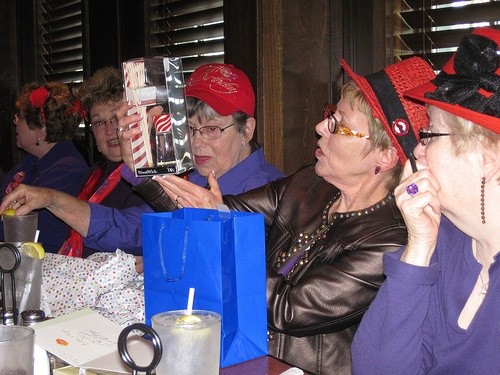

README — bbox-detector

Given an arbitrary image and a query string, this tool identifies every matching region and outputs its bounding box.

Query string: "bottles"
[0,306,44,326]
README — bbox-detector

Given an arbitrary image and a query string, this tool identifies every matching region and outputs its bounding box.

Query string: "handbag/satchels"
[141,208,269,368]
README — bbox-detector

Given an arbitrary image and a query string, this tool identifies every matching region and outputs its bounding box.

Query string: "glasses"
[15,112,27,122]
[89,116,118,131]
[188,119,246,140]
[419,127,458,146]
[323,103,389,148]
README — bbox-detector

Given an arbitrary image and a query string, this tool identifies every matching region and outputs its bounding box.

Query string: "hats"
[403,27,500,134]
[340,56,437,166]
[185,63,255,117]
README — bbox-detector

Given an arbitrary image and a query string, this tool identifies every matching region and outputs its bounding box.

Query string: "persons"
[54,65,144,259]
[352,27,500,375]
[118,56,435,375]
[0,81,91,254]
[0,62,285,275]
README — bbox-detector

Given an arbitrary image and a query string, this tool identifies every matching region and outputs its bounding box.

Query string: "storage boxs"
[121,56,196,178]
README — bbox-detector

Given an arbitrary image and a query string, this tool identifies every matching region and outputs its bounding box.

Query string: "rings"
[14,200,16,203]
[174,196,179,205]
[118,128,125,131]
[407,183,418,196]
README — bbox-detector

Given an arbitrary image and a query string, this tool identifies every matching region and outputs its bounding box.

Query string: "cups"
[0,240,42,313]
[3,212,39,244]
[0,325,35,375]
[150,310,222,375]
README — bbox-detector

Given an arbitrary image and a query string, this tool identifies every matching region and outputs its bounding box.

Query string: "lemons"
[21,242,45,259]
[170,315,207,334]
[2,208,16,215]
